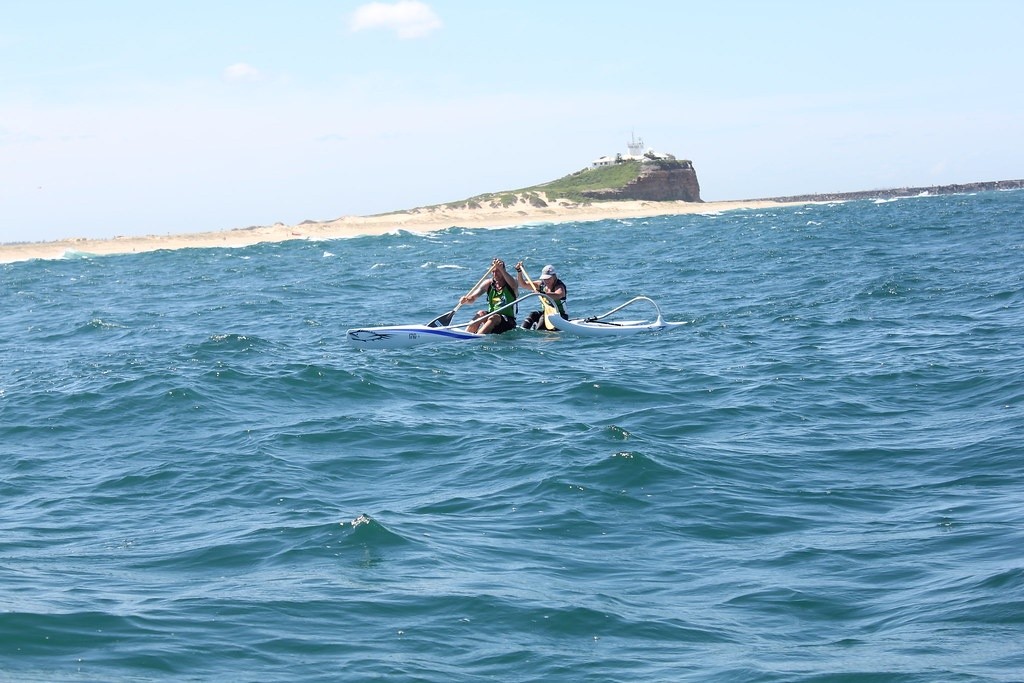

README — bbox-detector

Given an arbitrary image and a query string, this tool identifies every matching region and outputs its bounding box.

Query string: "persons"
[459,259,519,336]
[514,261,570,332]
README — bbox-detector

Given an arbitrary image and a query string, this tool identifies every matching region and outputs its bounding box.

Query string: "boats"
[345,293,688,352]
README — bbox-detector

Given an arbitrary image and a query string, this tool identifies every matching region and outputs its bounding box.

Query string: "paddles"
[584,315,623,326]
[427,262,495,327]
[520,264,559,330]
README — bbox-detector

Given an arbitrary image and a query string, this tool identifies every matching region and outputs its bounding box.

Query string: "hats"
[539,265,555,280]
